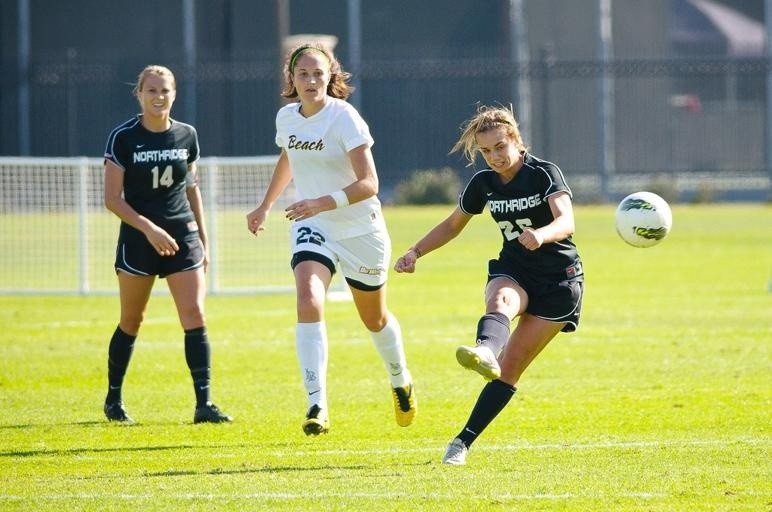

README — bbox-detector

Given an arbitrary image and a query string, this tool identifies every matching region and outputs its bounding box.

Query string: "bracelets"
[409,247,422,258]
[330,190,349,209]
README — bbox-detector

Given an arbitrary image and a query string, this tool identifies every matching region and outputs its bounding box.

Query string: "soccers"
[614,191,674,249]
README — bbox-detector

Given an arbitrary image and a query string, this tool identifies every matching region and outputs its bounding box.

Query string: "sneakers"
[389,368,417,428]
[301,403,331,434]
[103,396,134,423]
[193,402,232,424]
[455,343,503,381]
[441,437,468,466]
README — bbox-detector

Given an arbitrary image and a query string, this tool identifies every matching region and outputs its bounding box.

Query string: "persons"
[104,65,235,423]
[394,100,584,464]
[246,42,416,436]
